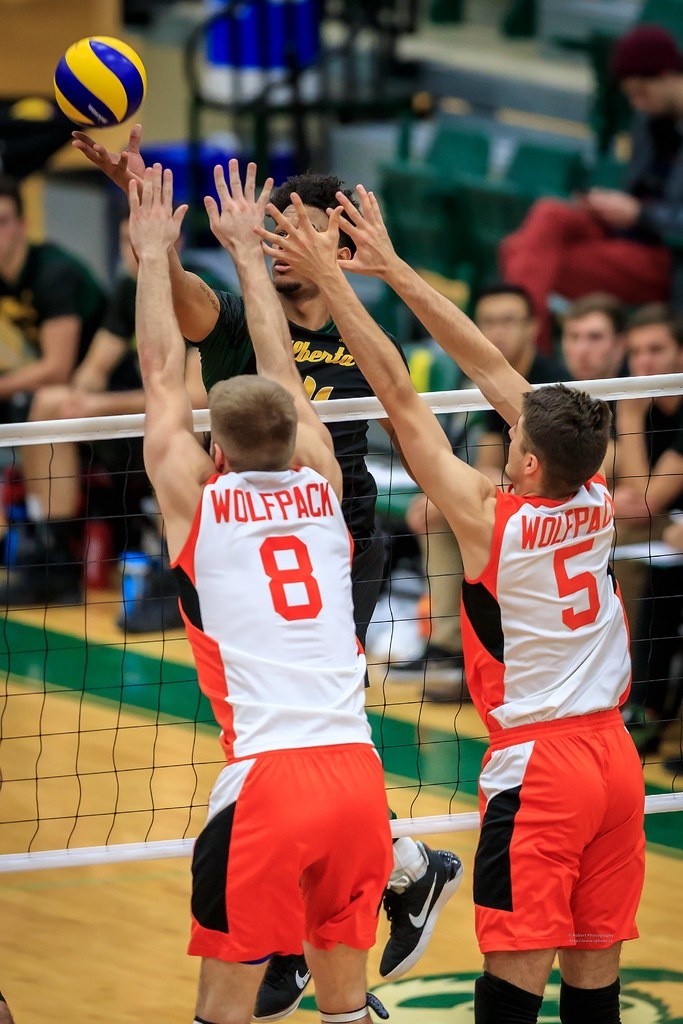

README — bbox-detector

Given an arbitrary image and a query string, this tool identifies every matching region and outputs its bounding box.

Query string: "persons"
[72,128,462,1020]
[504,26,683,359]
[405,281,683,772]
[252,184,646,1024]
[0,184,229,613]
[128,158,393,1024]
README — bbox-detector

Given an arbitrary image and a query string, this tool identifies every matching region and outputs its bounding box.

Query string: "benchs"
[186,0,683,524]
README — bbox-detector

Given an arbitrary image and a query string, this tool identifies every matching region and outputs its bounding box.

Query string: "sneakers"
[252,954,312,1022]
[380,840,463,979]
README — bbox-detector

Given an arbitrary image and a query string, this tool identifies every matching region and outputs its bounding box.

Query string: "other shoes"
[0,568,83,608]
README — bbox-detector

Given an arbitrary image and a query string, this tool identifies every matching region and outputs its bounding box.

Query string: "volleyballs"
[49,33,151,131]
